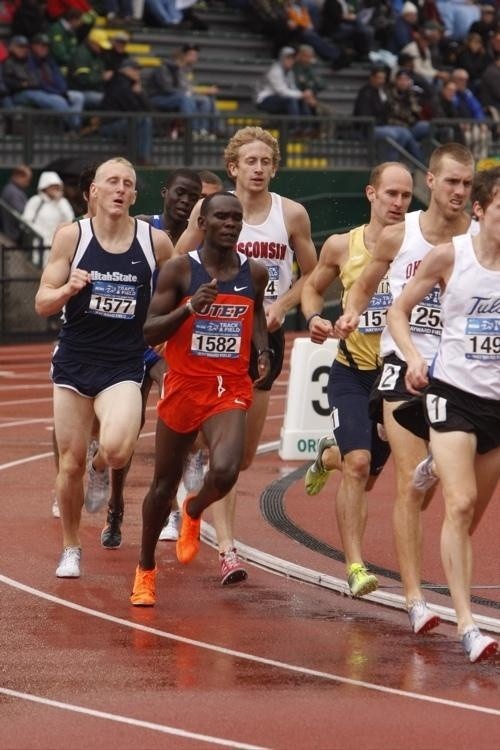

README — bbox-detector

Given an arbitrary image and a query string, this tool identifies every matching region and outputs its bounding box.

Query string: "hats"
[425,21,446,31]
[11,35,28,46]
[88,29,113,51]
[400,1,418,14]
[33,33,49,45]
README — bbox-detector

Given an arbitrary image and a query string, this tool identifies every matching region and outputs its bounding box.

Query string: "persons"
[171,126,318,586]
[143,42,219,142]
[130,191,273,607]
[300,160,415,597]
[0,166,84,265]
[101,168,203,549]
[157,170,227,541]
[334,143,480,636]
[0,2,154,165]
[293,43,336,140]
[254,47,313,137]
[386,167,500,664]
[34,157,175,578]
[244,0,450,101]
[52,165,99,519]
[433,1,499,116]
[353,53,487,165]
[145,1,211,34]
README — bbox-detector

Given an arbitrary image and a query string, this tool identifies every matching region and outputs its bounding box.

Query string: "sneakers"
[101,506,124,549]
[183,450,204,492]
[459,625,498,663]
[130,560,157,605]
[85,441,109,513]
[56,547,82,578]
[176,496,201,565]
[414,456,437,492]
[305,435,336,497]
[158,509,180,541]
[408,599,440,636]
[346,563,378,598]
[219,547,248,585]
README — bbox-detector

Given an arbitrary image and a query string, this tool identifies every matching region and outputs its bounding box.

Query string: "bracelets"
[306,313,320,331]
[186,297,196,314]
[257,347,276,362]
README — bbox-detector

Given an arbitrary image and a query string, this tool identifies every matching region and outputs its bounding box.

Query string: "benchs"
[128,0,367,113]
